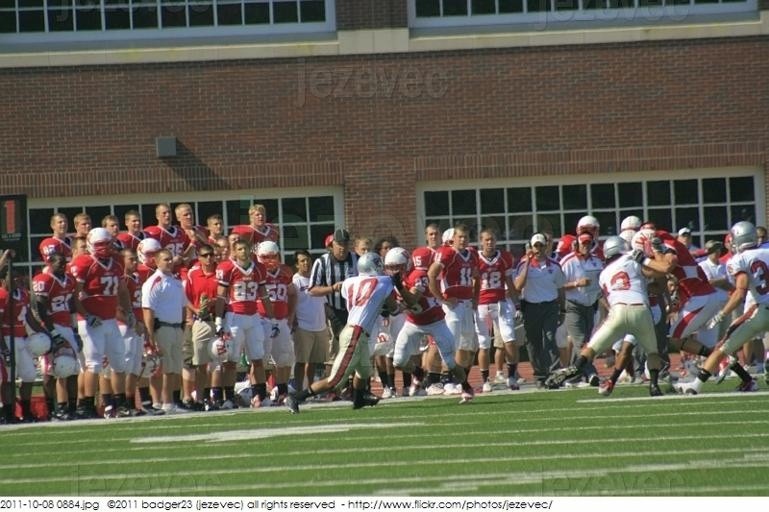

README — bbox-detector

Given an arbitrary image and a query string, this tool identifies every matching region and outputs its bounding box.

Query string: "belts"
[159,321,183,328]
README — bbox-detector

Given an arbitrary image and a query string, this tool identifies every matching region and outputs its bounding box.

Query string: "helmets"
[85,228,113,259]
[442,229,455,244]
[602,216,656,261]
[730,221,758,250]
[52,354,76,379]
[576,215,601,240]
[384,247,412,271]
[24,332,52,357]
[258,241,279,257]
[136,237,162,266]
[357,252,383,275]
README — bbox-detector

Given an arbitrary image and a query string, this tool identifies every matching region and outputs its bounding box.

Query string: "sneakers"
[250,391,289,408]
[545,366,614,396]
[483,374,527,392]
[328,385,395,410]
[620,369,701,396]
[715,354,764,392]
[50,392,239,420]
[288,391,299,413]
[402,376,475,404]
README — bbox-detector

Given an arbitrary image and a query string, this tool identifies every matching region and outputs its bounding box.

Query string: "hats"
[325,235,333,247]
[578,234,592,243]
[679,228,690,235]
[334,229,350,242]
[531,234,547,246]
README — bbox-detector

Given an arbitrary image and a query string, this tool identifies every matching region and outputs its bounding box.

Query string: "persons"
[2,201,768,424]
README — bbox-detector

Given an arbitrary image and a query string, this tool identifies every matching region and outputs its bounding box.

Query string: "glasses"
[200,253,214,258]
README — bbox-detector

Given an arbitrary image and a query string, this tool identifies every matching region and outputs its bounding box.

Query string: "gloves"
[705,311,727,328]
[1,349,14,367]
[84,313,102,328]
[127,311,136,329]
[53,334,72,348]
[73,328,83,352]
[706,242,723,253]
[388,271,403,291]
